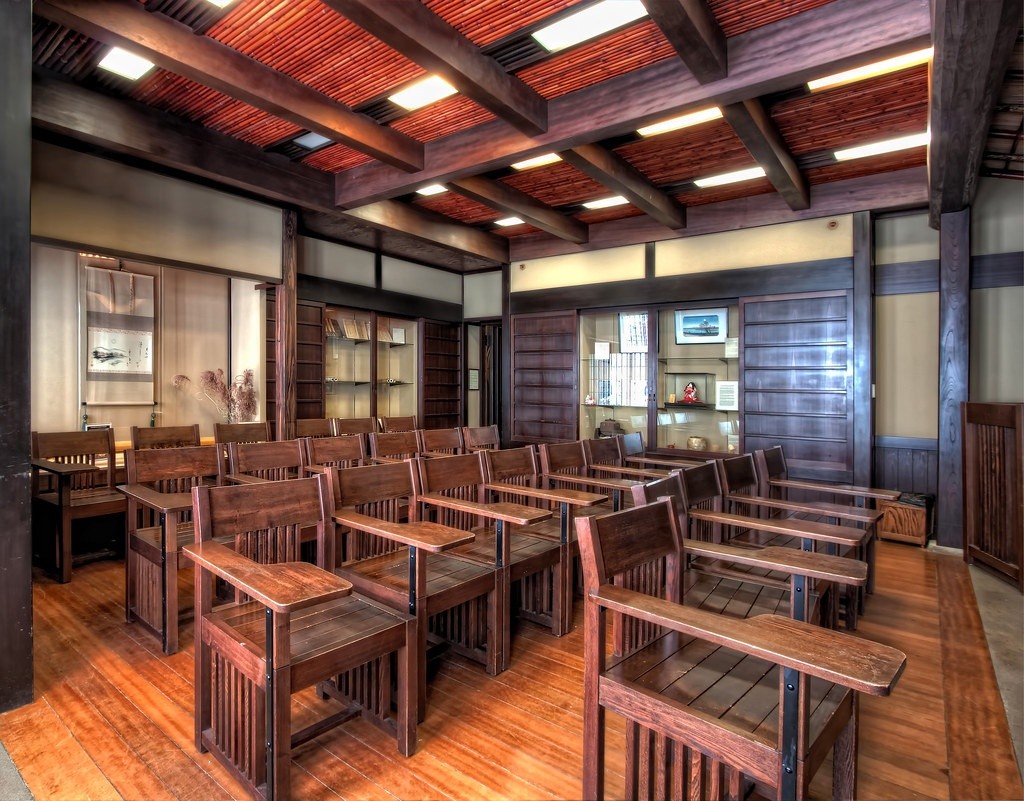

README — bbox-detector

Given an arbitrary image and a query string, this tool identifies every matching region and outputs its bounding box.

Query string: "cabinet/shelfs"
[326,306,371,419]
[652,297,739,463]
[579,303,652,458]
[376,315,417,418]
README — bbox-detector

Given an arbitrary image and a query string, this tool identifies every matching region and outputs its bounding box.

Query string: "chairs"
[716,452,885,617]
[671,459,870,633]
[182,473,418,801]
[227,439,328,484]
[323,458,503,723]
[756,445,903,597]
[297,415,417,438]
[31,428,127,584]
[631,472,869,631]
[484,431,708,637]
[129,423,201,450]
[115,443,227,657]
[416,449,554,673]
[305,424,501,475]
[213,421,267,445]
[574,495,909,801]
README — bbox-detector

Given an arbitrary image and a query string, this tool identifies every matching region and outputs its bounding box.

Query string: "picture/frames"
[674,306,729,345]
[468,368,479,390]
